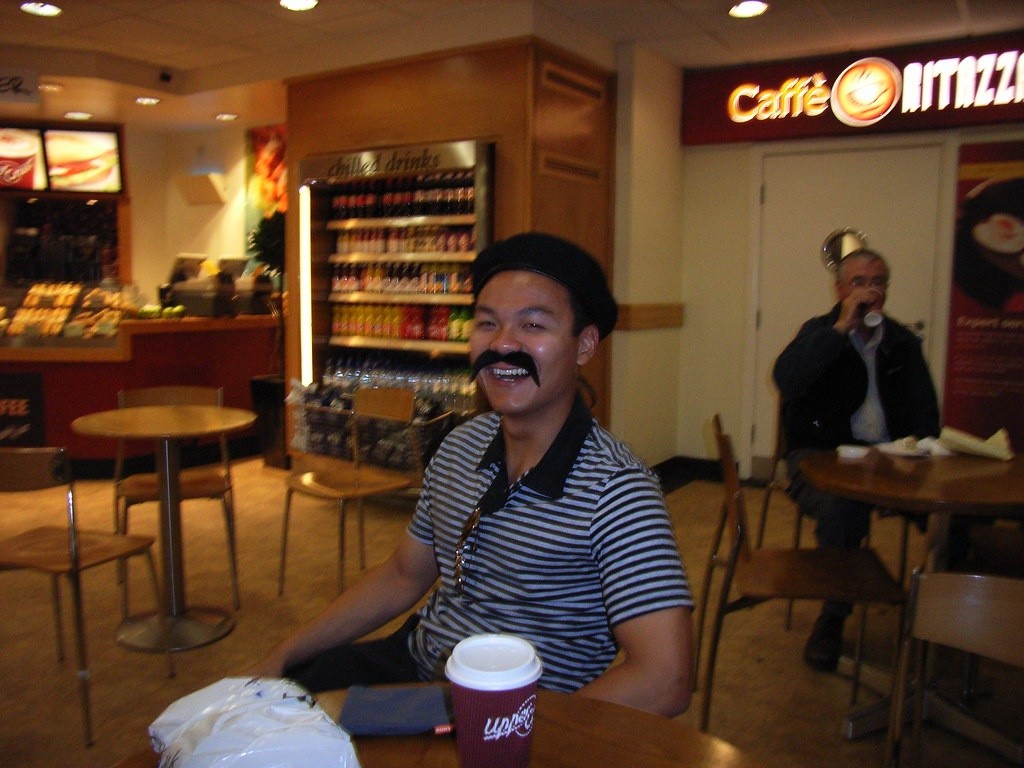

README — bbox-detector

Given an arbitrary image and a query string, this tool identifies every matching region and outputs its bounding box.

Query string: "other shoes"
[803,614,847,671]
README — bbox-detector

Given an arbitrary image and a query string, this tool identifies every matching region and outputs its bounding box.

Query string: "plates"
[974,223,1024,252]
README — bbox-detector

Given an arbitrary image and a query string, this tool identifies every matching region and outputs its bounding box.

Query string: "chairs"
[755,407,871,631]
[276,384,419,601]
[691,414,909,741]
[1,446,178,747]
[111,384,244,620]
[882,562,1024,768]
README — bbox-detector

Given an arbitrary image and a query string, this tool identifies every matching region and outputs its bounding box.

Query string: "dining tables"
[71,405,257,653]
[111,682,757,768]
[800,444,1024,739]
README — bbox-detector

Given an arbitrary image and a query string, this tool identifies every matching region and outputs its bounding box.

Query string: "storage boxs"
[0,281,132,345]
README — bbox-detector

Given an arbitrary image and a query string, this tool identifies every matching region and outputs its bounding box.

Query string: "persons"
[260,231,695,715]
[772,248,974,670]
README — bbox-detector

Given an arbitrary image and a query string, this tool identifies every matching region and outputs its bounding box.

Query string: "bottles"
[321,358,476,415]
[328,170,474,343]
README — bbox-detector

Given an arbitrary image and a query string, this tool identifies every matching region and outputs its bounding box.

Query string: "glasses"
[451,506,480,596]
[838,276,887,292]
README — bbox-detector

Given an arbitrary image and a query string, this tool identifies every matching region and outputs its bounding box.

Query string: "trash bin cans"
[249,376,289,468]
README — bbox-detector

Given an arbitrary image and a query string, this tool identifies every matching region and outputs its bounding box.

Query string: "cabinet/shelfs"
[293,137,494,478]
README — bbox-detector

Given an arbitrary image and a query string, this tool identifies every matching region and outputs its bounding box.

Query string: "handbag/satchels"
[149,676,360,768]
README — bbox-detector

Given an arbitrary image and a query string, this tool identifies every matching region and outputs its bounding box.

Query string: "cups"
[444,633,543,768]
[860,288,883,327]
[0,132,38,189]
[990,215,1020,239]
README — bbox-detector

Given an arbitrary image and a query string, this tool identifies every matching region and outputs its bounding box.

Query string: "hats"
[470,231,619,345]
